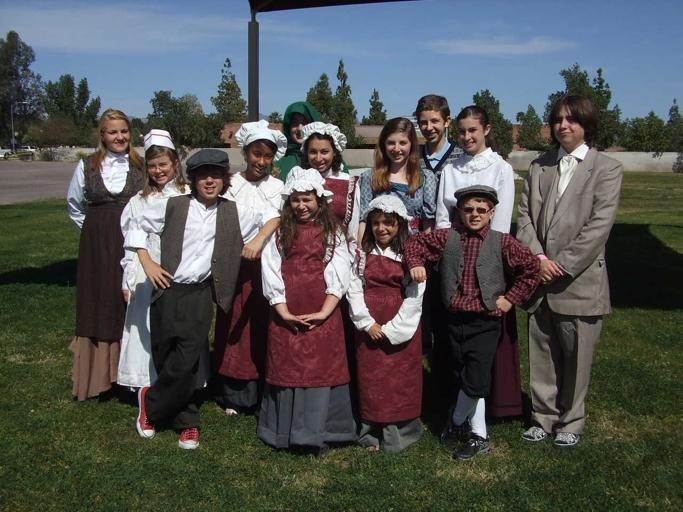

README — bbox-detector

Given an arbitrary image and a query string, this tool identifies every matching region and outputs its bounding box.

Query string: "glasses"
[463,207,492,214]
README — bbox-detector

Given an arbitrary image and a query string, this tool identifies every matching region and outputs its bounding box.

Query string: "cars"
[0,144,11,158]
[14,144,38,156]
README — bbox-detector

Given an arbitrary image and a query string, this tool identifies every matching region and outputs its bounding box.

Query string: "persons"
[64,108,146,403]
[254,164,358,450]
[121,149,245,449]
[340,194,428,454]
[354,115,438,247]
[290,120,360,267]
[412,95,466,175]
[515,93,626,447]
[215,119,285,416]
[268,101,350,180]
[117,127,191,407]
[403,182,542,461]
[433,105,526,423]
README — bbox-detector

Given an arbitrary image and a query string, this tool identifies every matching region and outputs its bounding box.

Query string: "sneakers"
[179,427,199,450]
[136,387,156,440]
[440,408,469,449]
[454,433,490,460]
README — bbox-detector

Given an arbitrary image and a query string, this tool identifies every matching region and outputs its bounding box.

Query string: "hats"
[142,119,414,224]
[454,185,499,206]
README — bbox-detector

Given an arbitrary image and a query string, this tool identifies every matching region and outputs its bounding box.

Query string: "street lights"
[10,101,27,152]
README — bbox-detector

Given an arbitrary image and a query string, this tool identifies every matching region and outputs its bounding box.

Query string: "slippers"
[522,426,548,442]
[554,432,580,447]
